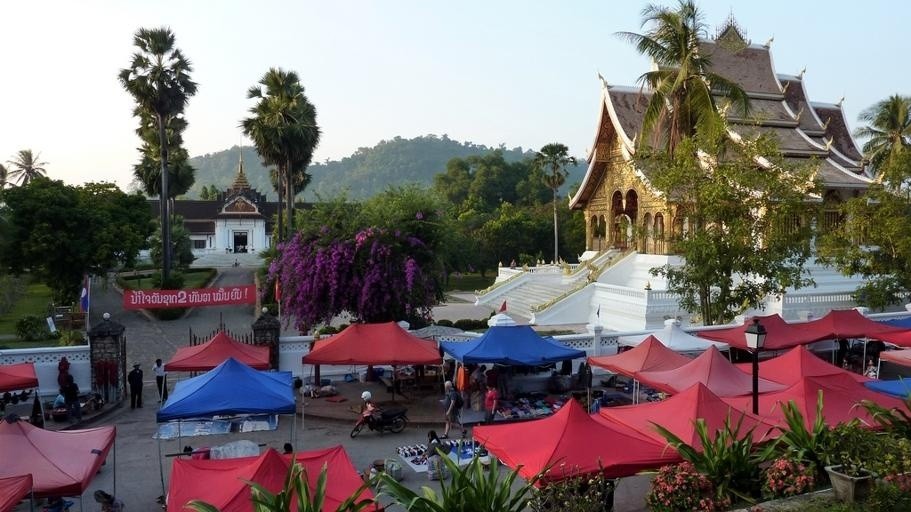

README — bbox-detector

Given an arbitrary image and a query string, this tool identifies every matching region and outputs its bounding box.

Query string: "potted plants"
[822,419,880,505]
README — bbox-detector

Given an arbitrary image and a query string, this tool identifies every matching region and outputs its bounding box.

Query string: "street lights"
[744,316,767,415]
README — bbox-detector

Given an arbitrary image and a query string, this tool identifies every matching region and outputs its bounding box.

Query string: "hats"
[133,361,140,366]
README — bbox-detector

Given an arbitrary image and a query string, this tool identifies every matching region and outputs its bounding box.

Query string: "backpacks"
[449,391,464,410]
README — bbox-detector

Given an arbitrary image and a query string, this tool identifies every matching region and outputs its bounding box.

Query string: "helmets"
[360,391,372,401]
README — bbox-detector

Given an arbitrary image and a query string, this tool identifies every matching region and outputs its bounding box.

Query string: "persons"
[152,358,167,403]
[127,362,143,409]
[421,430,446,480]
[437,380,467,439]
[52,392,67,423]
[235,244,246,252]
[864,359,878,379]
[282,442,292,456]
[842,358,852,372]
[470,364,486,413]
[483,383,499,425]
[59,374,82,423]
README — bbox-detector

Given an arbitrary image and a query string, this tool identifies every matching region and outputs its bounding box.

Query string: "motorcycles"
[350,398,408,438]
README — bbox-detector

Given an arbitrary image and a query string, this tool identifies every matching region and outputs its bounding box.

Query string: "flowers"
[523,454,817,510]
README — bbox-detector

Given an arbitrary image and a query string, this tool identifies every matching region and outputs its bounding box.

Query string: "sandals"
[462,429,467,438]
[438,434,448,439]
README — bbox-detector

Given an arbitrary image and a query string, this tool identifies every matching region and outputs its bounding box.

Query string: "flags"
[78,275,88,319]
